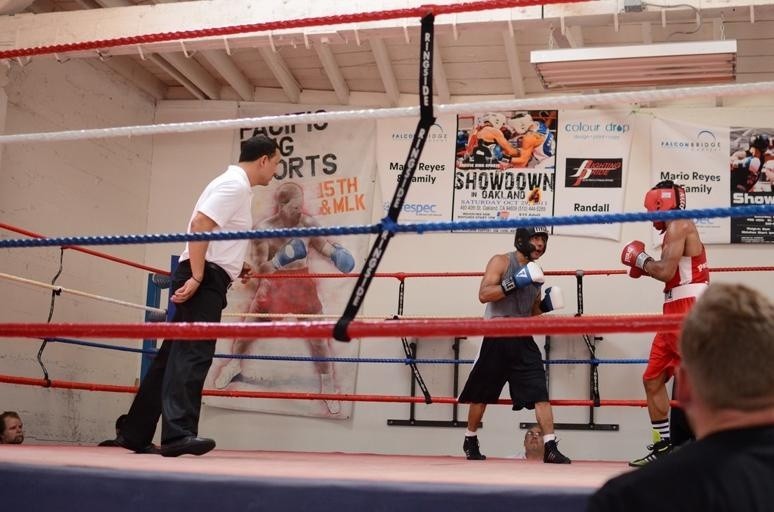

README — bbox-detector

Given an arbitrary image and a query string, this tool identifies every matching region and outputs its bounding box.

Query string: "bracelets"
[190,275,202,284]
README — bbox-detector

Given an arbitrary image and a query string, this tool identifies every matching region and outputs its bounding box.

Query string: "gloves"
[270,239,307,269]
[620,240,654,279]
[537,286,564,313]
[488,143,503,160]
[330,241,355,275]
[500,260,547,295]
[732,157,761,172]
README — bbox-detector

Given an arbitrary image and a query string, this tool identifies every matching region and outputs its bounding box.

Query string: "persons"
[470,114,517,167]
[454,218,573,464]
[96,414,159,448]
[0,411,25,443]
[518,423,546,462]
[120,135,282,456]
[213,181,354,415]
[586,284,773,512]
[617,181,710,470]
[493,112,556,171]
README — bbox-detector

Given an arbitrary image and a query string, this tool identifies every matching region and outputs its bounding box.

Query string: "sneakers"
[628,440,672,467]
[215,360,241,388]
[114,430,161,455]
[159,436,216,457]
[543,440,572,464]
[321,374,341,415]
[463,434,487,461]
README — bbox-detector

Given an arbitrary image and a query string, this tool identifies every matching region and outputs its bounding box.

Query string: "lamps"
[530,13,738,90]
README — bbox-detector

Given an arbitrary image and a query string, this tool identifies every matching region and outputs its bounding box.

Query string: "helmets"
[480,110,506,129]
[508,113,535,135]
[515,223,548,259]
[750,134,770,152]
[644,183,687,231]
